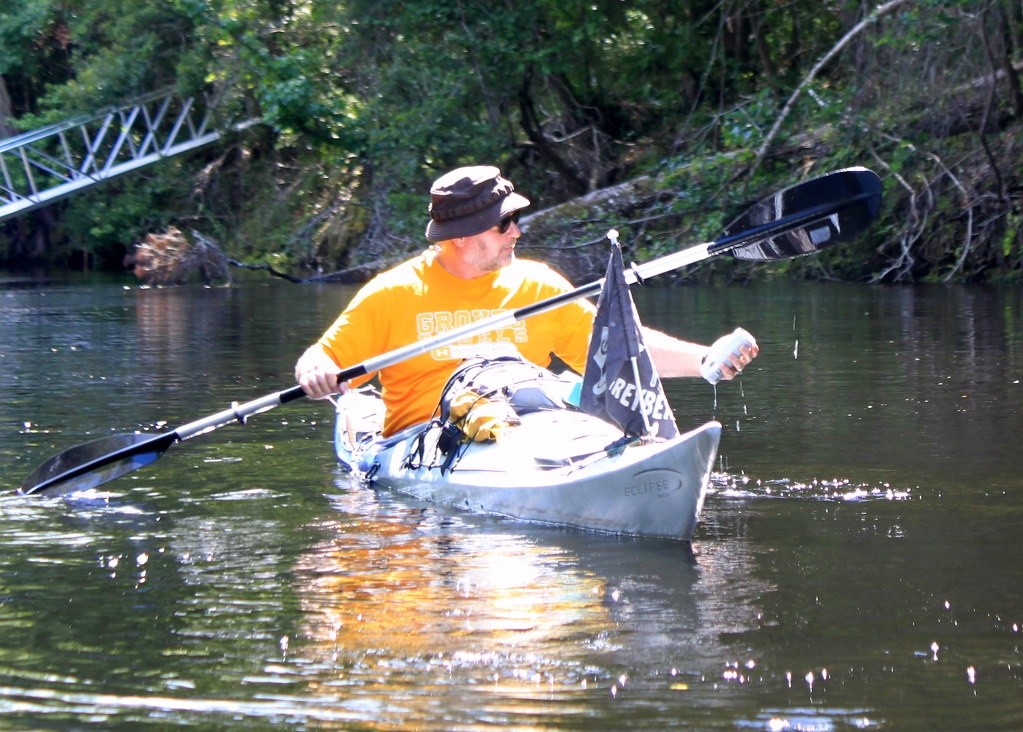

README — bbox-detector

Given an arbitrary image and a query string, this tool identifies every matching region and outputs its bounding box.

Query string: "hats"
[425,166,531,242]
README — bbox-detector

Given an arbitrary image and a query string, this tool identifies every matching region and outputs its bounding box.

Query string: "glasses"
[495,210,521,233]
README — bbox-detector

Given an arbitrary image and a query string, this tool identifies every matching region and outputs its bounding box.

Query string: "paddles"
[17,167,884,497]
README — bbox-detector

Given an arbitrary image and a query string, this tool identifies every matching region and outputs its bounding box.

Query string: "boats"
[334,382,723,542]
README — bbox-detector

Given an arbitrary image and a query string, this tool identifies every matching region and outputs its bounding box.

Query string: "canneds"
[698,327,756,385]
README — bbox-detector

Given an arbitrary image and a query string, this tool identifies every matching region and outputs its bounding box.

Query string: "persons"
[293,164,759,437]
